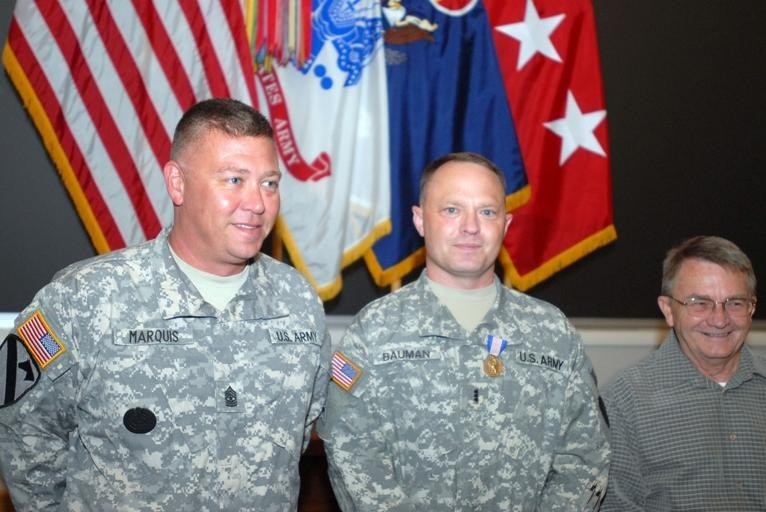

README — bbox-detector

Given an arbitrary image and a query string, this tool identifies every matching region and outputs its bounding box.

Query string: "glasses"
[673,294,755,316]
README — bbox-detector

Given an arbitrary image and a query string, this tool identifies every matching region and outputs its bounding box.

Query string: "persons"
[0,97,334,512]
[601,235,766,510]
[315,150,612,511]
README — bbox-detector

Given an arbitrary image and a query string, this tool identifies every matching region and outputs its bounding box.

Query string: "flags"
[0,0,619,302]
